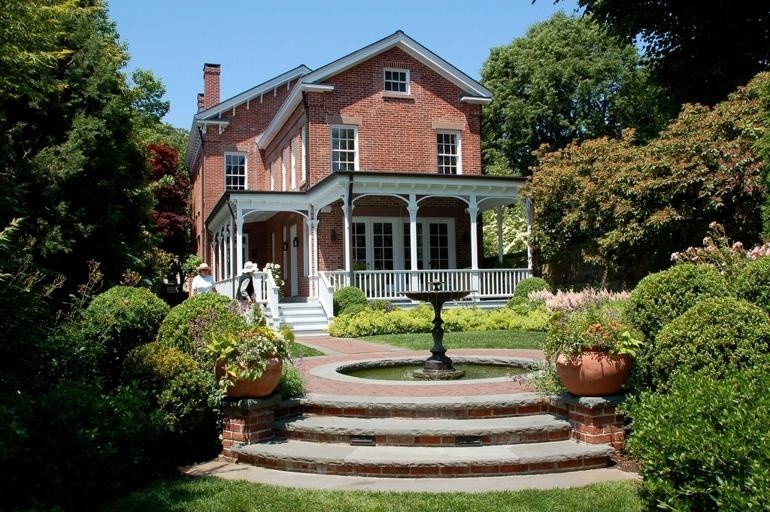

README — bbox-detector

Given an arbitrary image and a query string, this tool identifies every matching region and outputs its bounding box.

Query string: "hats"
[196,263,212,272]
[240,261,257,274]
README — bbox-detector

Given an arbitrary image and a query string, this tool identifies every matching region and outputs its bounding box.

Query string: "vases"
[211,357,282,398]
[554,345,628,398]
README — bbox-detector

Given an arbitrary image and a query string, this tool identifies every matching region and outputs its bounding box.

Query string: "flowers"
[184,309,296,398]
[539,298,647,364]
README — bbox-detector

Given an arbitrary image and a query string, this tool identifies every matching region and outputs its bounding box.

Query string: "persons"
[189,263,218,297]
[235,259,259,319]
